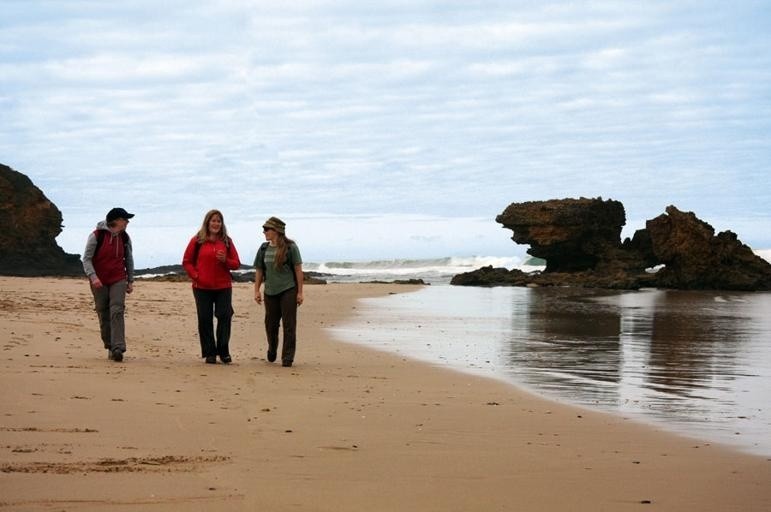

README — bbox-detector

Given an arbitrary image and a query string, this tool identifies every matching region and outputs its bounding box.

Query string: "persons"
[182,209,240,363]
[82,207,134,361]
[252,217,302,367]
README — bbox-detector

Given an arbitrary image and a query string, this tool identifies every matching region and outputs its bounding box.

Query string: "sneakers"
[268,350,276,362]
[109,349,123,361]
[283,360,292,367]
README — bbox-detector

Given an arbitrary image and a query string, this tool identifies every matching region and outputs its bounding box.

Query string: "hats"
[263,216,286,234]
[107,208,135,220]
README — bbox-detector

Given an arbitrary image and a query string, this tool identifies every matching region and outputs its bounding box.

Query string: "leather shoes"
[205,355,216,363]
[220,355,232,362]
